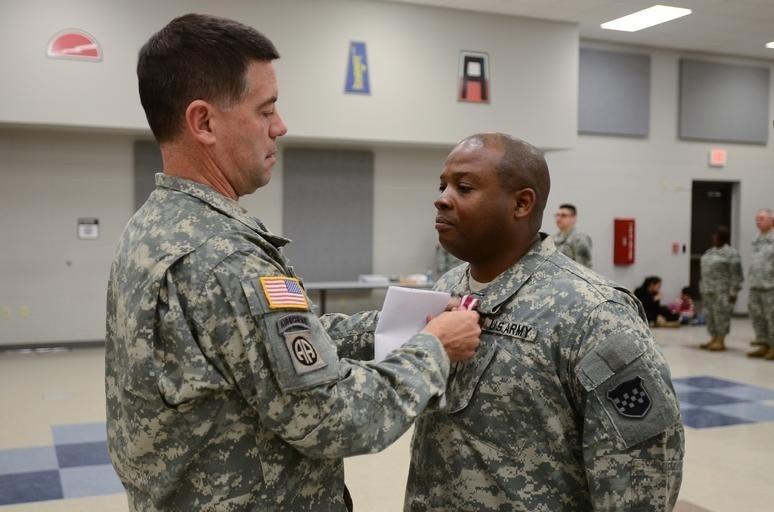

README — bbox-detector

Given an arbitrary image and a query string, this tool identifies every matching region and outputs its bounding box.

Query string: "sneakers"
[701,336,774,359]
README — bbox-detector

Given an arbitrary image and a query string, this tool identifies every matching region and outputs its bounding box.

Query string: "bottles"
[426,267,434,288]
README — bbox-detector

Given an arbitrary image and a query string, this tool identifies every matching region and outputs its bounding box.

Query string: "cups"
[389,275,399,283]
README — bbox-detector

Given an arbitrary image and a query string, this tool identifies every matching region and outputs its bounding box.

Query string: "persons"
[403,133,685,512]
[106,13,482,512]
[746,207,774,360]
[634,276,680,327]
[699,225,744,351]
[672,287,697,324]
[552,204,593,269]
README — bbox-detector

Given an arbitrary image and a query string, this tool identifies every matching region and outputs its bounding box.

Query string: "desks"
[303,279,434,315]
